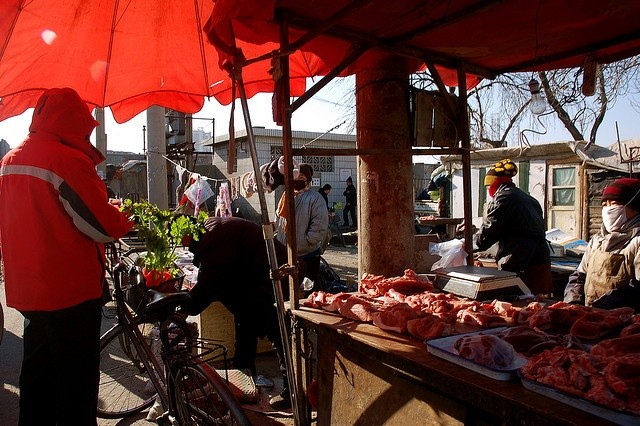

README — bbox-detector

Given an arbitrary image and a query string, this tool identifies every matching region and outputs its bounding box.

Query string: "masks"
[602,189,640,233]
[294,177,312,191]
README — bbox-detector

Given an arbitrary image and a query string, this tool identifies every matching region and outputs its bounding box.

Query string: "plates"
[477,257,496,263]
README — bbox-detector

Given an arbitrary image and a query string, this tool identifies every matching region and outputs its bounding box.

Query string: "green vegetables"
[119,197,210,279]
[332,201,342,214]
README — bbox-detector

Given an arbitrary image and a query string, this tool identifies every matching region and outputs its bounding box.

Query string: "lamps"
[528,2,546,115]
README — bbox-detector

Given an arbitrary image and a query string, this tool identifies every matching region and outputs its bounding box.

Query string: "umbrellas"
[0,0,482,426]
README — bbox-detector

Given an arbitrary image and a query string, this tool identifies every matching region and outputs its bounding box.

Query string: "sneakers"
[269,387,292,410]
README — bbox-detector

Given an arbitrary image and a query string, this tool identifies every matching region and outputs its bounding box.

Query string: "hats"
[484,159,518,187]
[298,163,313,182]
[346,176,352,182]
[601,178,640,212]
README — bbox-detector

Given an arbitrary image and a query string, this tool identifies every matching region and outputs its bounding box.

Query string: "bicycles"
[328,202,346,248]
[97,242,250,426]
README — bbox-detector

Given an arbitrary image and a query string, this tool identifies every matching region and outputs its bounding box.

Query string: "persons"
[162,216,295,410]
[564,178,637,309]
[341,175,358,230]
[270,162,329,296]
[0,87,133,425]
[318,184,332,203]
[473,159,552,298]
